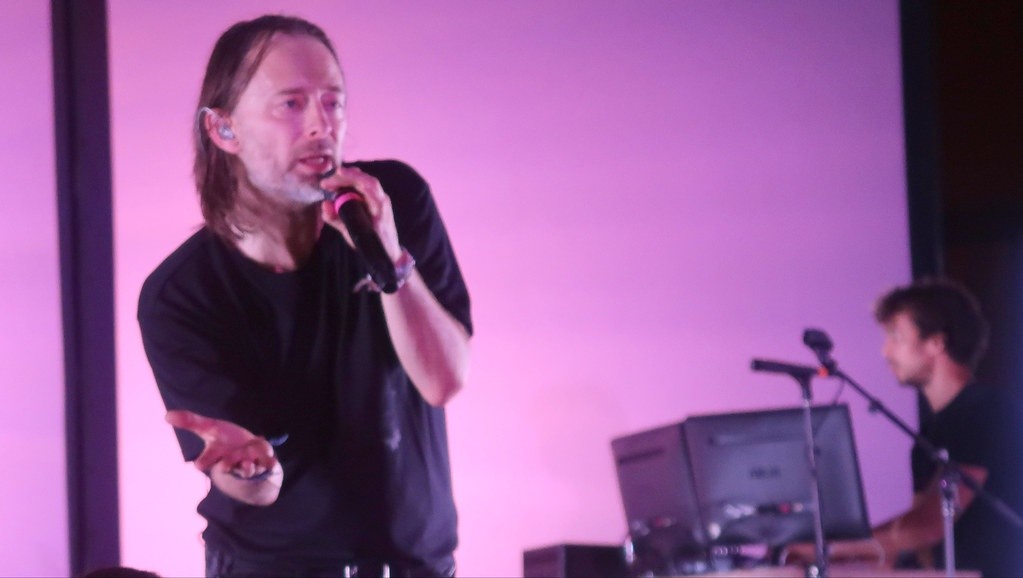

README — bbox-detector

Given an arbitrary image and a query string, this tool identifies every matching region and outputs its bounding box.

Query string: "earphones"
[218,124,235,140]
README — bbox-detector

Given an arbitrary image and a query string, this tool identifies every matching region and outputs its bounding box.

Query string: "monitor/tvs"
[609,402,873,578]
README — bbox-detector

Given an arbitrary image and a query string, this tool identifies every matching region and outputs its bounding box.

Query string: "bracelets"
[370,247,416,294]
[229,433,288,482]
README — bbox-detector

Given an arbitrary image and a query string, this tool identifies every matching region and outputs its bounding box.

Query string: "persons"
[791,278,1023,578]
[138,15,474,578]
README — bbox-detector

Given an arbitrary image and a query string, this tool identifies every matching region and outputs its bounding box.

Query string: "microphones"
[322,167,399,294]
[749,359,836,379]
